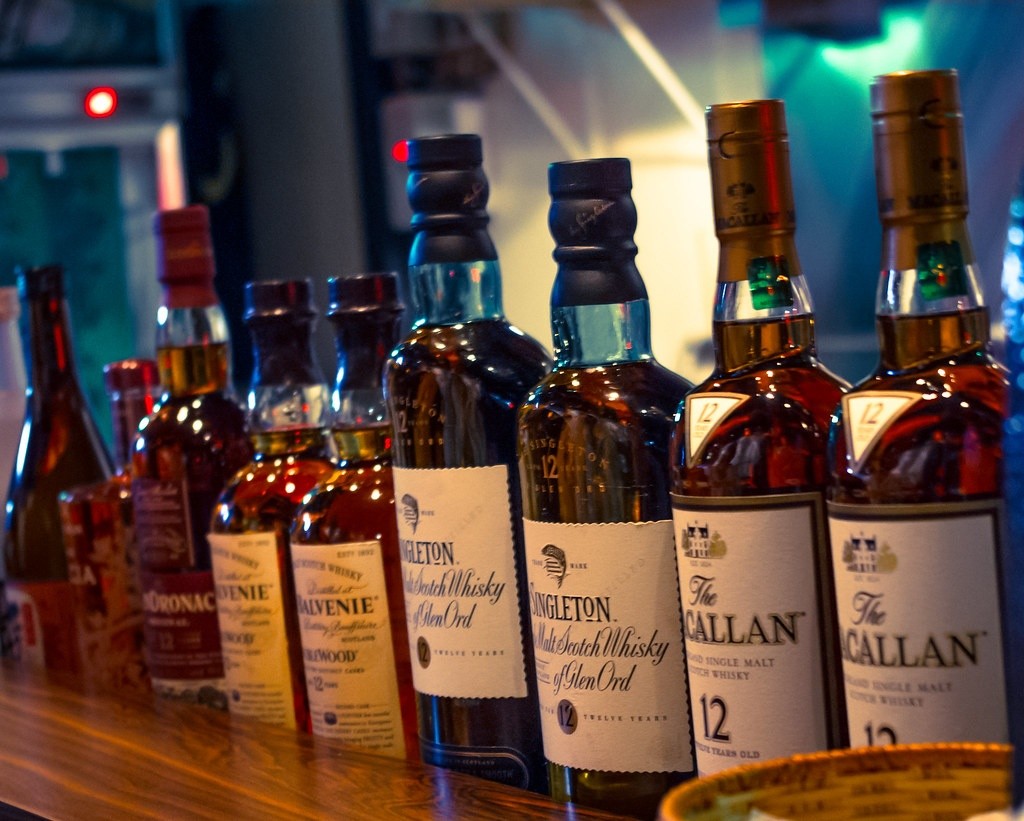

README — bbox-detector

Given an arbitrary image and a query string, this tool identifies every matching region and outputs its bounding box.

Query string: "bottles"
[1,68,1024,805]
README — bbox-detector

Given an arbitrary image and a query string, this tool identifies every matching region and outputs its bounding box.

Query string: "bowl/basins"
[661,742,1022,821]
